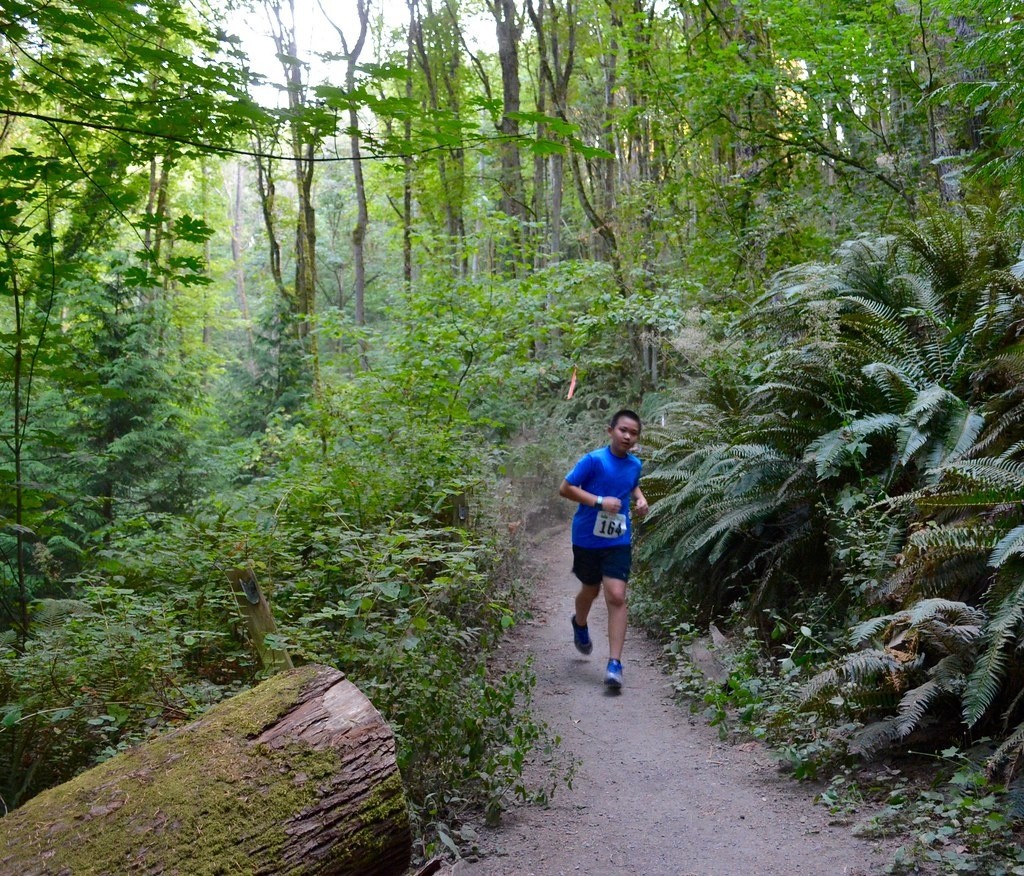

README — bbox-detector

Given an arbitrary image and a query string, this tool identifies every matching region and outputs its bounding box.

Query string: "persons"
[558,410,648,687]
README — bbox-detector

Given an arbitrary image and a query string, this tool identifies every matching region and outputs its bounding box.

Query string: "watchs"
[594,495,603,510]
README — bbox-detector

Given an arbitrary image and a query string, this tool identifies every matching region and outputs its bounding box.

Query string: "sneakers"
[572,614,592,655]
[604,660,622,688]
